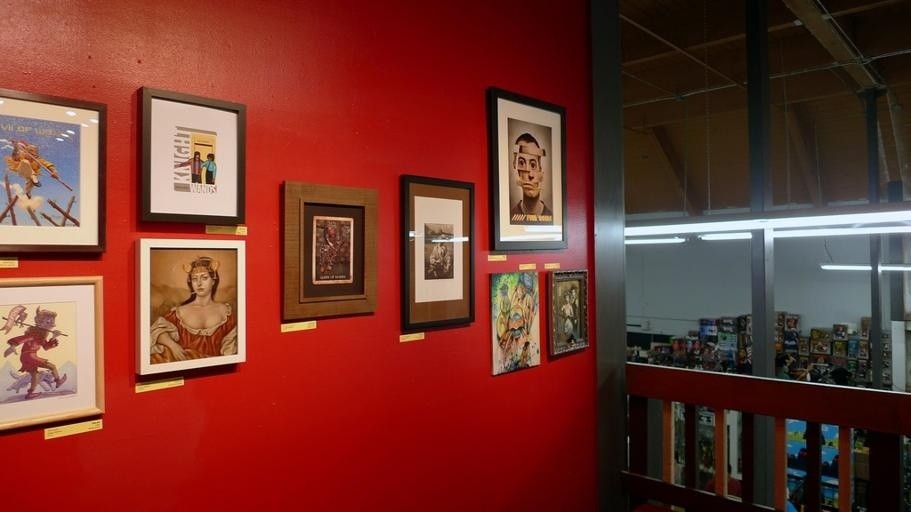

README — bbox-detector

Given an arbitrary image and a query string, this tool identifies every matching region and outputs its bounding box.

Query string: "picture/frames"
[138,84,249,226]
[279,178,380,323]
[398,173,477,332]
[486,86,570,253]
[134,235,248,376]
[546,267,590,358]
[0,86,107,258]
[0,274,107,434]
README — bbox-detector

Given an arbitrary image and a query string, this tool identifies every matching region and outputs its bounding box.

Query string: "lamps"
[624,49,911,272]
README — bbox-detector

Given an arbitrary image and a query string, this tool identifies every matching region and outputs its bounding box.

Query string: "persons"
[560,292,582,343]
[568,285,579,342]
[704,463,741,497]
[150,256,237,364]
[511,131,552,215]
[176,151,203,183]
[201,154,217,185]
[834,326,845,336]
[787,487,804,512]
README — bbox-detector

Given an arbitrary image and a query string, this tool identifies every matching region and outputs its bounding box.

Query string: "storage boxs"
[645,310,910,512]
